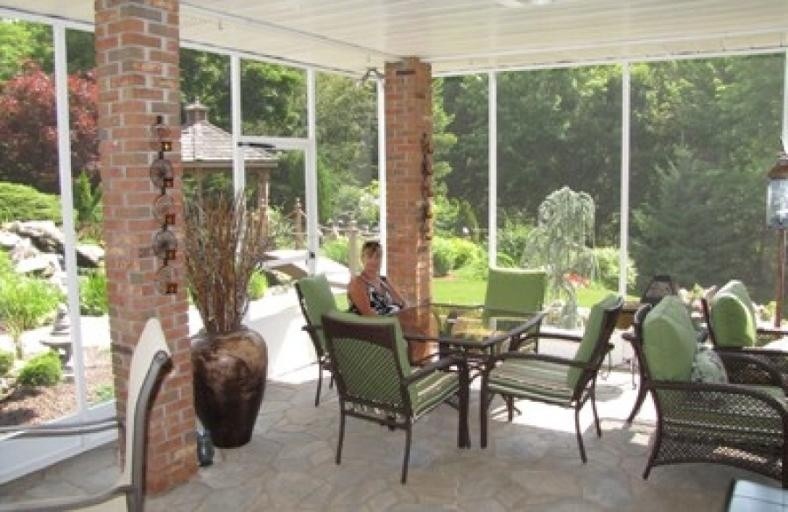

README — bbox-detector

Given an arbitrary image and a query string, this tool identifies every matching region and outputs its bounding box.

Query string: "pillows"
[690,348,728,400]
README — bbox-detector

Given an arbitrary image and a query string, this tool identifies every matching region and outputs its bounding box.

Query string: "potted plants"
[185,188,278,449]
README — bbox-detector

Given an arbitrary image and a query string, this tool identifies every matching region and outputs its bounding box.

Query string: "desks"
[385,304,548,449]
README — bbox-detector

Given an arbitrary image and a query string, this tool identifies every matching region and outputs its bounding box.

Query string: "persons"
[349,242,408,318]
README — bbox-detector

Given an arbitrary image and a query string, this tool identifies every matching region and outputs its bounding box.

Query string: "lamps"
[764,159,788,327]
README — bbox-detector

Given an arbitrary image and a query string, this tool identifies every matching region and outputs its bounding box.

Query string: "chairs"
[321,310,463,485]
[294,273,339,406]
[484,267,548,356]
[700,278,788,384]
[633,302,788,489]
[2,315,175,512]
[481,295,624,463]
